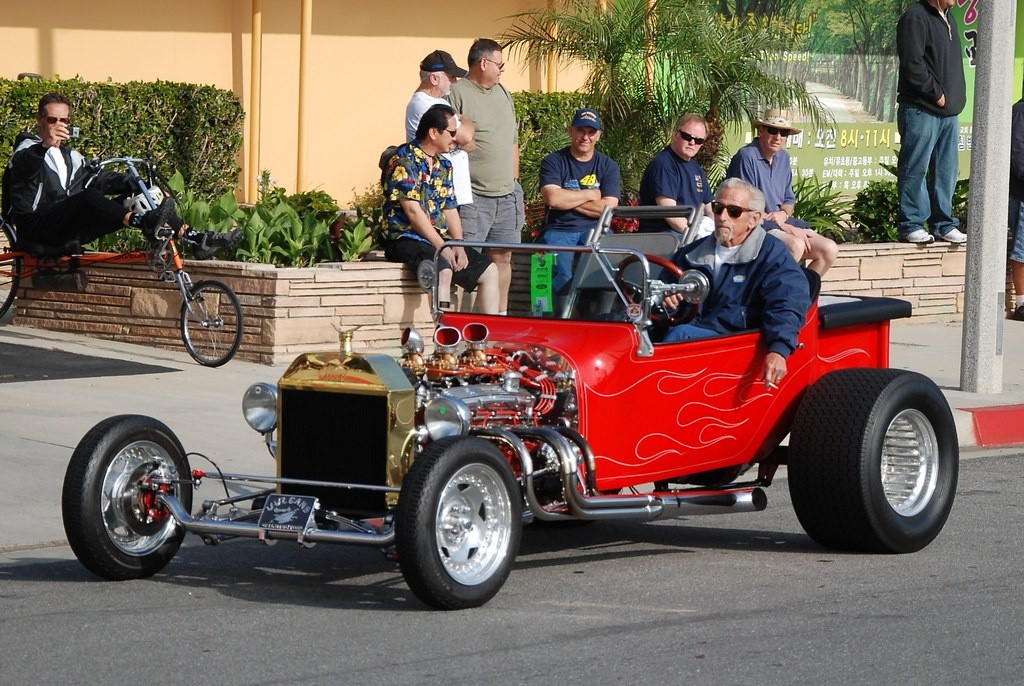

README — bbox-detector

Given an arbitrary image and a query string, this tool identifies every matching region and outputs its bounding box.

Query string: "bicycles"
[1,157,244,368]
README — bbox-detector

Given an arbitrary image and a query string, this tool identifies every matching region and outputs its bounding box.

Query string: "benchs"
[549,268,821,336]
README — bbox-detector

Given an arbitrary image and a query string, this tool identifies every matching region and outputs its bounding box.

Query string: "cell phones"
[65,127,80,138]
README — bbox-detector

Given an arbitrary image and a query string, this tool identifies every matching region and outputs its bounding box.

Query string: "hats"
[573,108,600,130]
[419,50,468,78]
[379,142,409,185]
[752,109,801,135]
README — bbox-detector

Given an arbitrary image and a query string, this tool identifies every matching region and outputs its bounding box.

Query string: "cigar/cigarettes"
[769,382,778,388]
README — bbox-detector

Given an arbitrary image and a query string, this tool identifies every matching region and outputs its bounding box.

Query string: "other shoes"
[1013,306,1024,322]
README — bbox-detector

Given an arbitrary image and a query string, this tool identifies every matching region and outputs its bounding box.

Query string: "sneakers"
[906,229,934,244]
[190,229,245,260]
[934,227,967,243]
[140,196,175,245]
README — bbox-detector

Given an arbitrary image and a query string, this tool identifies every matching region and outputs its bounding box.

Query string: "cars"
[60,205,956,603]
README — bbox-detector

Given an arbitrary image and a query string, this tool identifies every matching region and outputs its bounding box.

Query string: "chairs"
[559,203,706,319]
[1,132,83,257]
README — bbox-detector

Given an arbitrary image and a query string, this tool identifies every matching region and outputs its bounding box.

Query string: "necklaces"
[421,148,435,158]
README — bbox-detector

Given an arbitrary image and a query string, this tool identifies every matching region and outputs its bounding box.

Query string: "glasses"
[763,125,791,137]
[40,114,70,125]
[479,59,505,70]
[711,201,753,219]
[675,129,704,145]
[444,129,456,137]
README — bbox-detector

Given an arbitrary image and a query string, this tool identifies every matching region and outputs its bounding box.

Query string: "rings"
[776,378,781,380]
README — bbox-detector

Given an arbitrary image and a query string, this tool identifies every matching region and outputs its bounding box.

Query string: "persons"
[441,38,525,316]
[385,104,499,325]
[896,0,968,243]
[404,50,475,218]
[726,109,839,278]
[630,178,812,389]
[539,108,620,290]
[2,93,243,260]
[637,113,715,245]
[1008,99,1024,321]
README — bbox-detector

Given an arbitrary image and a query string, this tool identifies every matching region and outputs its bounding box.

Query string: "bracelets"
[780,209,790,219]
